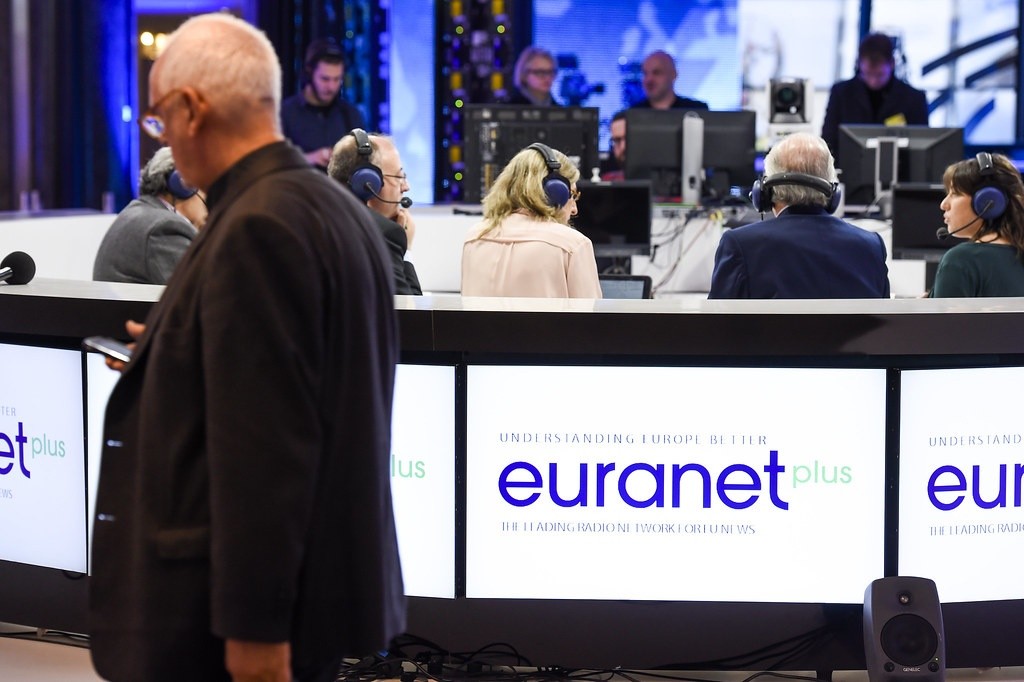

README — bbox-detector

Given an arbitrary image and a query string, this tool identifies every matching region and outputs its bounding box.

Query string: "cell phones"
[84,336,132,363]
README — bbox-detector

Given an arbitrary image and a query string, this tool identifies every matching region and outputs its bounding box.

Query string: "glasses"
[136,89,203,139]
[527,69,557,77]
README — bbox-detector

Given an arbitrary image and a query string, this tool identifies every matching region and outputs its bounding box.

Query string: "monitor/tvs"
[568,180,652,276]
[626,107,757,208]
[463,104,600,196]
[891,184,972,263]
[837,125,964,220]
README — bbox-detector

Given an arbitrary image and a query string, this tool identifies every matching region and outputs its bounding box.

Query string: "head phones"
[347,128,384,201]
[749,173,841,214]
[970,152,1009,220]
[528,142,572,209]
[168,167,198,199]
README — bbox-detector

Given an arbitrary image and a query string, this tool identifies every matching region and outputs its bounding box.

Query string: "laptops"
[598,274,651,299]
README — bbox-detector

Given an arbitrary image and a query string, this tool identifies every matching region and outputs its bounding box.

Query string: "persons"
[83,12,410,682]
[495,46,558,106]
[327,128,423,296]
[817,32,927,157]
[460,143,603,300]
[279,40,362,172]
[629,51,708,112]
[707,133,889,298]
[928,151,1024,297]
[603,112,628,171]
[94,147,208,284]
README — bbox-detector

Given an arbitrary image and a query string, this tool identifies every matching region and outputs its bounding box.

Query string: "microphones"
[371,188,412,208]
[936,208,986,240]
[0,251,36,285]
